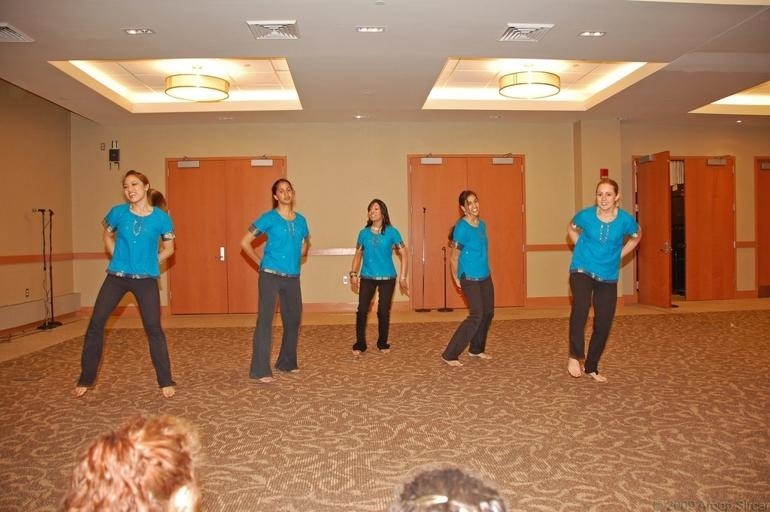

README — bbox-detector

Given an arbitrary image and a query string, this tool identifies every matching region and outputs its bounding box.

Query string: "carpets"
[1,307,768,512]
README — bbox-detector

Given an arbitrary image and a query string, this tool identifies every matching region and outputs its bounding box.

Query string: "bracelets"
[351,275,357,278]
[350,271,359,273]
[401,276,407,279]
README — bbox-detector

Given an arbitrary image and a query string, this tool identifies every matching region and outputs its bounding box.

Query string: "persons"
[63,411,200,512]
[349,198,409,358]
[565,180,644,385]
[440,190,495,366]
[240,179,311,384]
[72,170,176,399]
[389,459,512,511]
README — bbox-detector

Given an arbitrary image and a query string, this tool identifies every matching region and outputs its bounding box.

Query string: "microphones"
[32,208,45,212]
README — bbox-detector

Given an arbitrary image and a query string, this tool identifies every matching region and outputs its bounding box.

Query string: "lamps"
[498,71,561,99]
[164,74,230,102]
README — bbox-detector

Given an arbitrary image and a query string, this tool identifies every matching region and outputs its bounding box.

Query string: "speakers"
[109,148,120,161]
